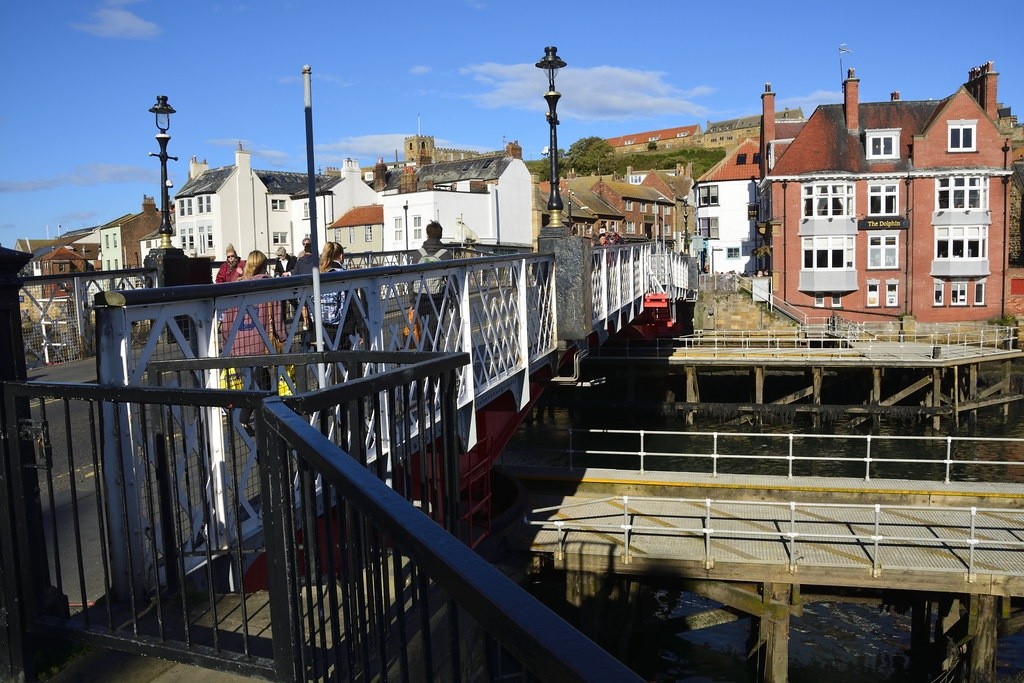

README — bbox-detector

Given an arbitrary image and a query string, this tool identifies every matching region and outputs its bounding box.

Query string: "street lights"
[535,46,569,229]
[147,95,181,252]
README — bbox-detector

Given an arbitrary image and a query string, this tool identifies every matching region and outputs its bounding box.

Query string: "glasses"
[227,255,235,258]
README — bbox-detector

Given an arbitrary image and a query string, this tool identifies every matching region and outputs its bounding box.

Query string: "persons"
[591,228,625,246]
[404,222,457,355]
[219,236,374,438]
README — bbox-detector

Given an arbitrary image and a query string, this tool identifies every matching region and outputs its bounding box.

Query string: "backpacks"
[309,268,345,326]
[413,246,448,294]
[403,309,422,350]
